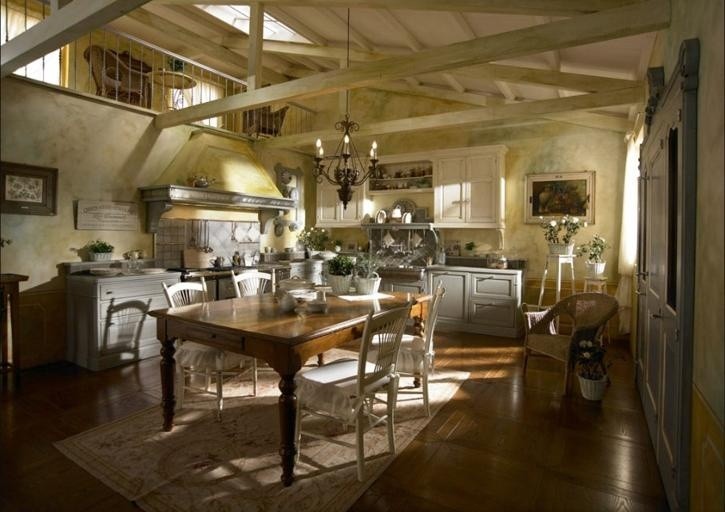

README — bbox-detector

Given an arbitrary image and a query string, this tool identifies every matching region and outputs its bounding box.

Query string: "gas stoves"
[168,266,239,272]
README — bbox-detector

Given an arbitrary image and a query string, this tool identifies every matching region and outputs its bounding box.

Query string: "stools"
[583,276,610,346]
[537,257,577,310]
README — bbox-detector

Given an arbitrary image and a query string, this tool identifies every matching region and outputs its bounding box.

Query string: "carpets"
[52,347,470,512]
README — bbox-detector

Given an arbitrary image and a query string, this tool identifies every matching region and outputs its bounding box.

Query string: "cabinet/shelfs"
[315,145,508,227]
[65,257,523,373]
[634,38,701,511]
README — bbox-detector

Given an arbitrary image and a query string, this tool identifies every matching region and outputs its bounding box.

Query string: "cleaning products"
[438,247,446,264]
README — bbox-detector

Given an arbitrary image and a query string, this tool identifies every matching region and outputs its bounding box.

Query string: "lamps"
[312,91,379,210]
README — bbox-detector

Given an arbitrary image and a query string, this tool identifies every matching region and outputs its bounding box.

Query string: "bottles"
[127,251,141,272]
[486,252,499,267]
[499,256,508,268]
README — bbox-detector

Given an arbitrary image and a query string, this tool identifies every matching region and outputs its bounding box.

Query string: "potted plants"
[464,241,476,256]
[86,239,114,262]
[576,234,611,274]
[545,214,580,255]
[575,339,611,403]
[299,226,328,258]
[333,239,343,252]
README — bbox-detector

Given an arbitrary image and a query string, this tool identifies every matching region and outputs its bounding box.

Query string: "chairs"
[160,268,445,482]
[84,44,153,107]
[242,105,290,138]
[520,293,619,397]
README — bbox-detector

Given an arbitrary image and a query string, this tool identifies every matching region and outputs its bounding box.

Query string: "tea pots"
[192,172,217,188]
[210,256,232,270]
[227,252,243,267]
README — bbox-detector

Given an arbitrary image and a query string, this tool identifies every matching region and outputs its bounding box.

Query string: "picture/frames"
[1,160,59,217]
[523,171,596,226]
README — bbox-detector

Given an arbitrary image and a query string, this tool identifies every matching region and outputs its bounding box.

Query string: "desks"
[1,273,29,398]
[146,70,197,112]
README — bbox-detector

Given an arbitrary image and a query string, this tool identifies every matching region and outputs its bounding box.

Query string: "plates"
[89,267,121,277]
[375,211,386,225]
[141,267,166,274]
[392,199,415,221]
